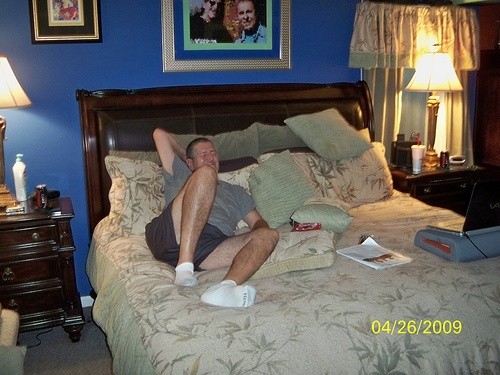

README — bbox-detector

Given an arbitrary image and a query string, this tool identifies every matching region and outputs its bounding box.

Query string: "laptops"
[425,179,500,238]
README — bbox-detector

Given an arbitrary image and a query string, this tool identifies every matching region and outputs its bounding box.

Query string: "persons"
[190,0,233,43]
[146,128,280,306]
[235,0,267,45]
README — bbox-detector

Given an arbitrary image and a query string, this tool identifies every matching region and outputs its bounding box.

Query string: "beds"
[76,79,500,375]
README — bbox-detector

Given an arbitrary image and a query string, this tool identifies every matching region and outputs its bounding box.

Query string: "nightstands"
[0,196,86,342]
[389,162,485,211]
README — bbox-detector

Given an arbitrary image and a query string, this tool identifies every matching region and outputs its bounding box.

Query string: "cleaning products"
[12,153,28,202]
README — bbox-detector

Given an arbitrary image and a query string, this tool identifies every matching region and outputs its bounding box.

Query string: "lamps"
[0,54,32,210]
[402,52,464,171]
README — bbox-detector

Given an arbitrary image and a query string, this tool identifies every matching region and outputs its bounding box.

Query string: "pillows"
[103,108,394,279]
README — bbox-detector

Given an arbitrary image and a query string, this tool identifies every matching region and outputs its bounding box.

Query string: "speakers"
[394,147,407,165]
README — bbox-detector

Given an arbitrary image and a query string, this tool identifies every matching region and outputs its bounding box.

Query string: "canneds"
[35,184,49,208]
[440,151,448,168]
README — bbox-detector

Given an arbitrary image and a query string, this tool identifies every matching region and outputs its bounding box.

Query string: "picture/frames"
[161,0,291,72]
[28,0,103,44]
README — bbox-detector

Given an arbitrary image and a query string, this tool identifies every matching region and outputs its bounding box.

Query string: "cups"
[411,145,425,174]
